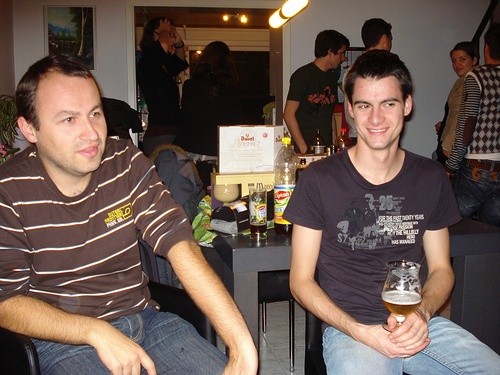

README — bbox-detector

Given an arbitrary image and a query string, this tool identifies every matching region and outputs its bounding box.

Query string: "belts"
[472,160,500,171]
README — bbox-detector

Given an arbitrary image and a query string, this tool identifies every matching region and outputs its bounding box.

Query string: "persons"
[182,40,237,155]
[282,49,500,375]
[137,18,190,144]
[342,17,394,146]
[431,39,480,191]
[449,30,500,228]
[284,29,350,155]
[0,53,259,375]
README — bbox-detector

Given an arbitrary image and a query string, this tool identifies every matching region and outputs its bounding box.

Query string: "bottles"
[296,159,307,182]
[335,128,351,153]
[274,137,299,234]
[310,128,327,154]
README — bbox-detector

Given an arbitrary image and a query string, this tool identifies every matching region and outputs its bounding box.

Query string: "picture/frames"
[43,5,97,72]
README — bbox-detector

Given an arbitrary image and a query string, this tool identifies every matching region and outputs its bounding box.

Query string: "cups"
[249,189,268,240]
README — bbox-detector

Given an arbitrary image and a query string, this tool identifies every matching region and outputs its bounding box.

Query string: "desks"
[203,213,500,361]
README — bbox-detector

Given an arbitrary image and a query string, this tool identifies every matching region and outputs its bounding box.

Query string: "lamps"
[269,0,309,29]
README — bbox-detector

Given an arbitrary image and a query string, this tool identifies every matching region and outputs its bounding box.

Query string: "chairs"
[210,270,295,372]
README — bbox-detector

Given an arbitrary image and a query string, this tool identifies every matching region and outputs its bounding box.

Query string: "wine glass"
[382,260,423,332]
